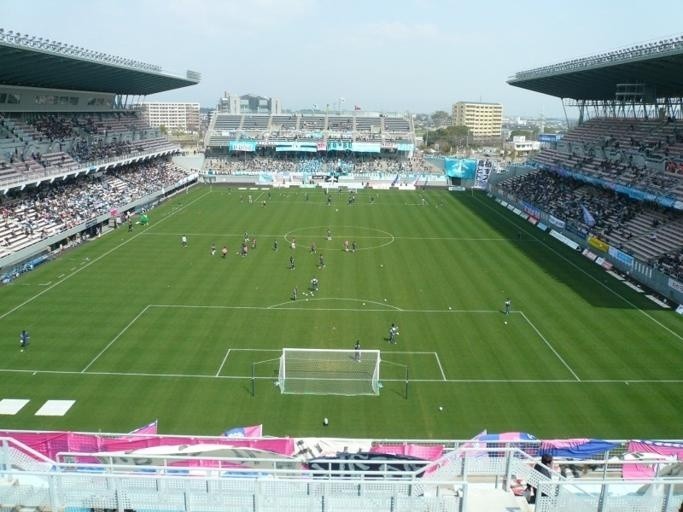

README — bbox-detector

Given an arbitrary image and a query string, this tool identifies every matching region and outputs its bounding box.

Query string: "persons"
[0,112,683,363]
[530,453,557,505]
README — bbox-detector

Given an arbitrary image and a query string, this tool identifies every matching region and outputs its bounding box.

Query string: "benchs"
[209,115,411,143]
[0,114,192,258]
[497,117,682,277]
